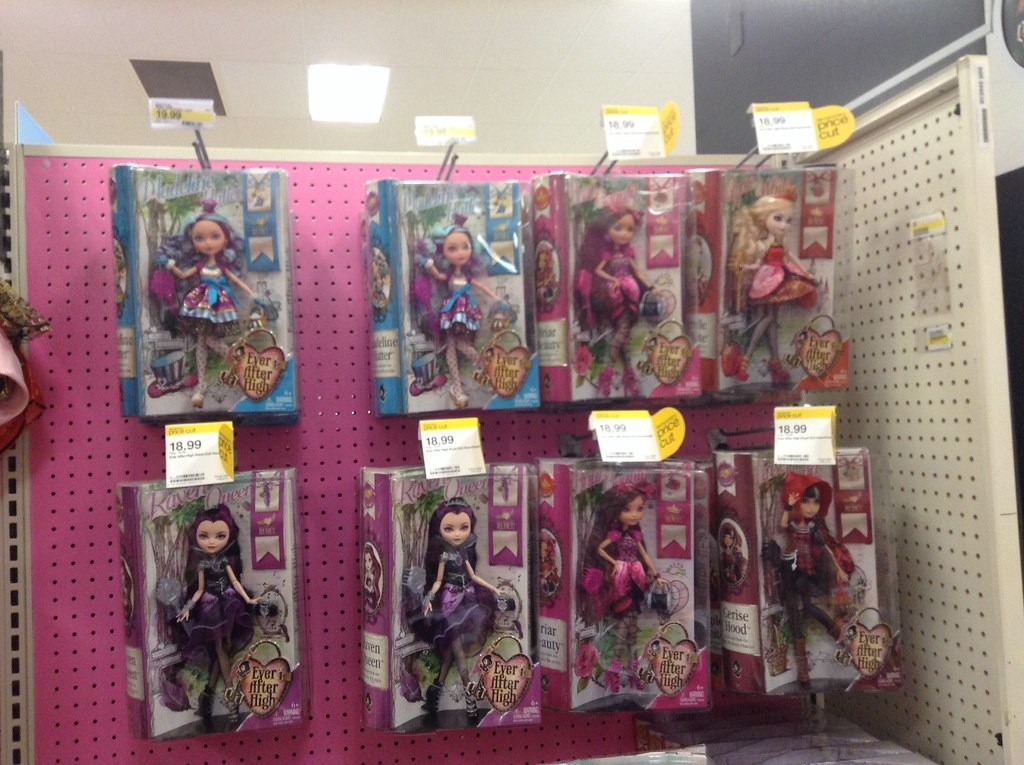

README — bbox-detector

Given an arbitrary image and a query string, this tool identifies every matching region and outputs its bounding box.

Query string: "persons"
[416,497,506,727]
[578,206,661,396]
[584,483,668,693]
[416,214,508,405]
[173,504,265,733]
[161,200,271,407]
[774,471,855,690]
[732,195,820,382]
